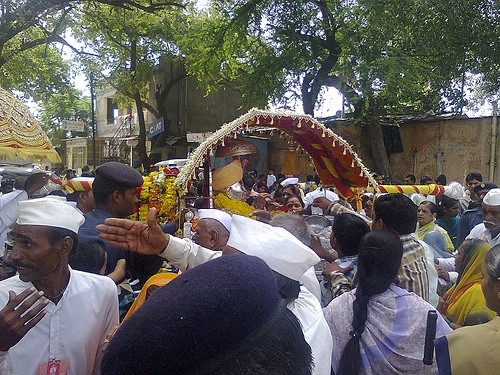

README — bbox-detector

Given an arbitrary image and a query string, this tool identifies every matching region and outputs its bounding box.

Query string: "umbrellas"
[217,139,257,159]
[0,86,63,164]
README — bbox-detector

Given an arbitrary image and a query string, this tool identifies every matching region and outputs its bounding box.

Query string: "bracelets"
[327,269,343,279]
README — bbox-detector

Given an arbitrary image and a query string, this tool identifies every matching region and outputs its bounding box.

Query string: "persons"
[436,237,496,330]
[320,210,370,308]
[0,162,190,318]
[374,169,499,250]
[435,244,500,375]
[435,188,500,291]
[415,201,454,257]
[98,207,330,375]
[191,166,340,258]
[0,195,120,375]
[311,192,441,312]
[322,229,455,375]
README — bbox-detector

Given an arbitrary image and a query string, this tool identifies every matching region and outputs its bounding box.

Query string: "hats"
[197,209,232,233]
[95,162,144,188]
[483,188,500,206]
[15,199,86,234]
[100,254,287,375]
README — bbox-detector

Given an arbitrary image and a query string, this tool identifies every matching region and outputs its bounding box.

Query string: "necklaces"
[39,285,68,300]
